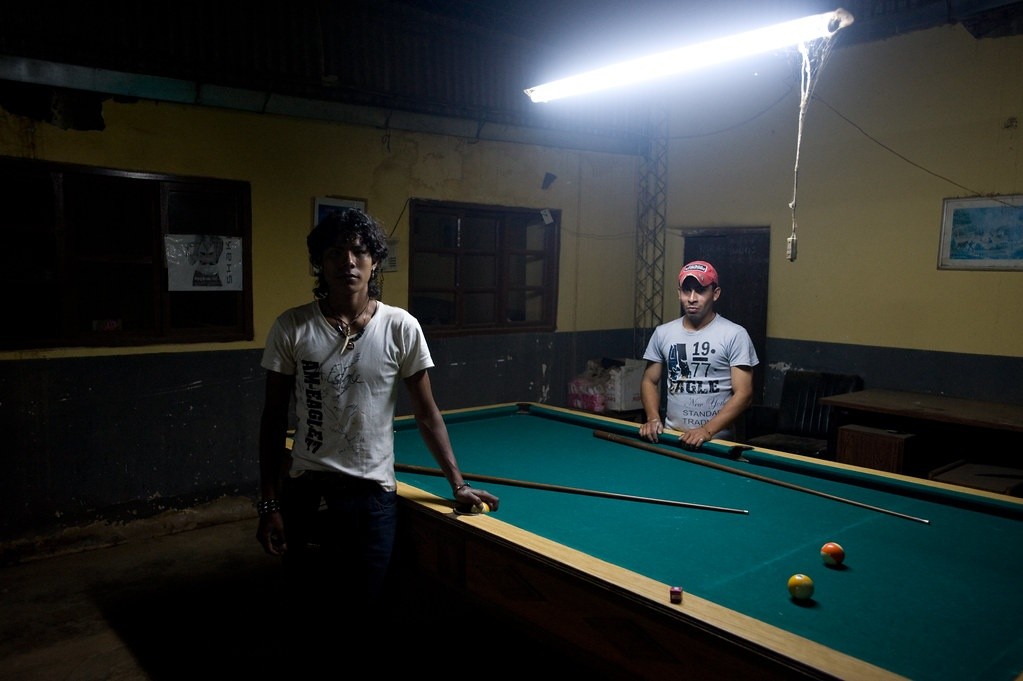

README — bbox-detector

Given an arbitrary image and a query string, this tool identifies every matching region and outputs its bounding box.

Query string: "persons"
[639,260,759,448]
[184,235,224,286]
[255,207,499,621]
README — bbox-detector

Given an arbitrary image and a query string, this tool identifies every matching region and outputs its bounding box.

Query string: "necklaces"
[326,297,369,356]
[683,313,715,330]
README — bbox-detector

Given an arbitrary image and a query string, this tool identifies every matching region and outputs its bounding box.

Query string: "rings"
[700,438,703,442]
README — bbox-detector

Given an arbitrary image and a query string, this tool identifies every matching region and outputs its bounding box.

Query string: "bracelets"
[453,481,471,495]
[257,499,282,518]
[701,425,712,437]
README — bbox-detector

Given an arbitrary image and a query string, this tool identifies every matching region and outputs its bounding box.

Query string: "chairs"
[745,370,846,462]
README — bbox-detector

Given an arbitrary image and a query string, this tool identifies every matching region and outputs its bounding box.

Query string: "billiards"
[819,541,846,567]
[788,573,814,599]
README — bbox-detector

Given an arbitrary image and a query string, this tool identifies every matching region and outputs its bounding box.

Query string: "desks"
[815,387,1023,497]
[276,402,1023,681]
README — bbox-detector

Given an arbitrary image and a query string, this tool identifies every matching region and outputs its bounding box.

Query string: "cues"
[393,462,750,514]
[591,430,929,525]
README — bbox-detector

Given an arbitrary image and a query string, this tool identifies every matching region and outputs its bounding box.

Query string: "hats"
[679,261,718,287]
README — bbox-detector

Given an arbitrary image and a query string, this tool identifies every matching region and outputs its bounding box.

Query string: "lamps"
[523,8,854,103]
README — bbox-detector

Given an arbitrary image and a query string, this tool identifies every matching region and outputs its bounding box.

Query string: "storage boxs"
[588,355,648,410]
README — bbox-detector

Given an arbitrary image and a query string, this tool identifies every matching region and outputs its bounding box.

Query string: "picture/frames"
[936,192,1023,272]
